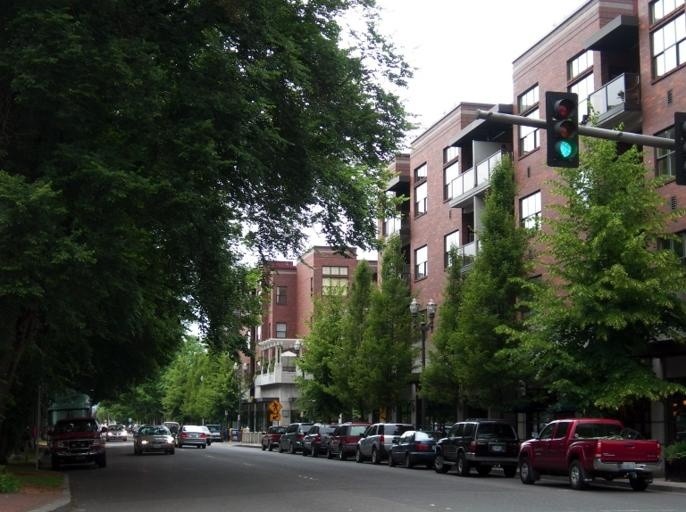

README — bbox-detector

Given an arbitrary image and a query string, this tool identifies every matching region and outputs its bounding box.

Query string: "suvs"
[47,417,108,469]
[261,419,520,479]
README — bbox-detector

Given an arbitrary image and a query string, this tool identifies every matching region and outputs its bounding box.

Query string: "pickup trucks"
[520,416,662,490]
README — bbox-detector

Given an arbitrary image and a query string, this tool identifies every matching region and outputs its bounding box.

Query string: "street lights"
[409,296,437,430]
[293,339,307,417]
[232,361,248,430]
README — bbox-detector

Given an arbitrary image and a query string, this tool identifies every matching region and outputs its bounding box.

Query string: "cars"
[98,421,226,454]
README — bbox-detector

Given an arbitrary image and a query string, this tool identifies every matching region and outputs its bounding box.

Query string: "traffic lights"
[545,90,581,169]
[675,112,686,185]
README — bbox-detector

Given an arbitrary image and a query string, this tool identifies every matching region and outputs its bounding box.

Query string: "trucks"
[42,407,94,455]
